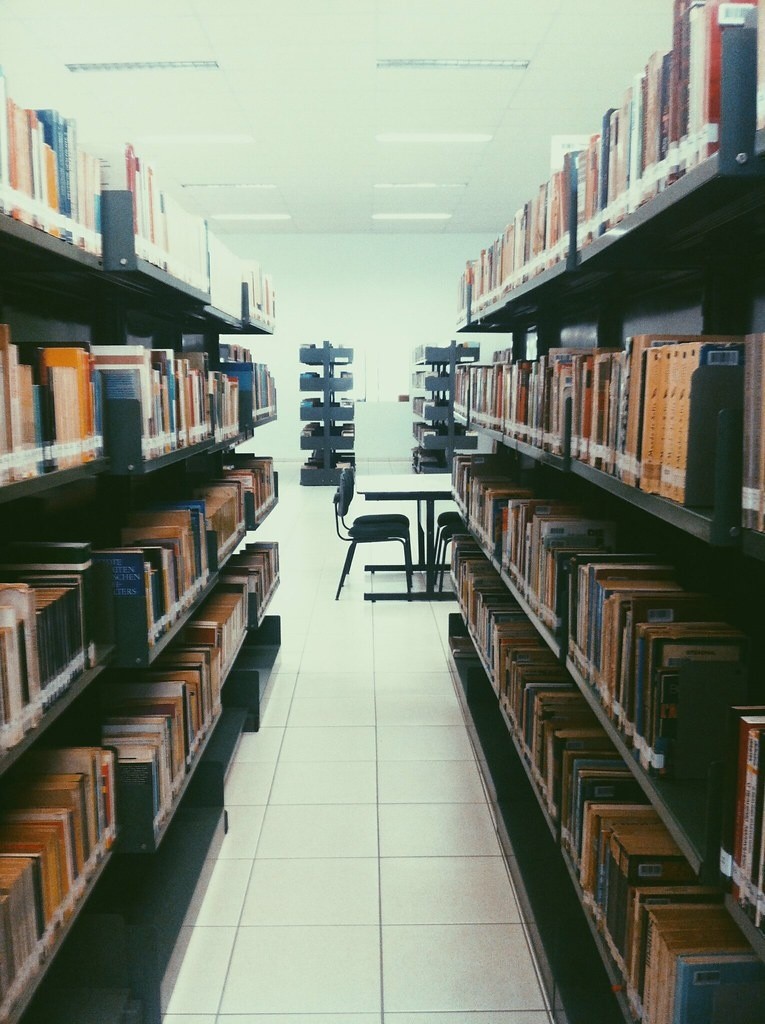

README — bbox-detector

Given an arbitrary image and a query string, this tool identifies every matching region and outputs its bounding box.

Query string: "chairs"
[332,466,473,602]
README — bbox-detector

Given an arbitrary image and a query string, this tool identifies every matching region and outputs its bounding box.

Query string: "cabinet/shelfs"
[0,0,763,1024]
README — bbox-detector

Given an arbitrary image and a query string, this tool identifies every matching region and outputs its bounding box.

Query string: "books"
[451,335,765,1024]
[0,457,280,1009]
[459,0,765,327]
[298,341,483,477]
[1,98,277,457]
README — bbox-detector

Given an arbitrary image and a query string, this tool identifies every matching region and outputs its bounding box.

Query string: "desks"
[355,472,457,603]
